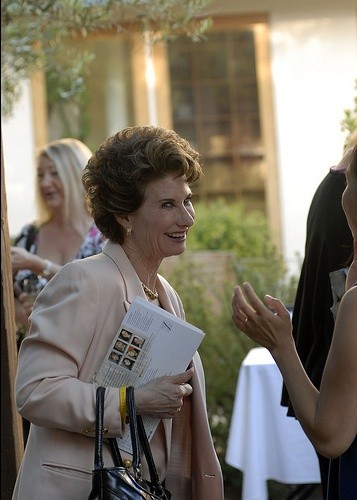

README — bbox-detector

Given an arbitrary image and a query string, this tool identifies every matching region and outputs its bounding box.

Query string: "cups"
[23,278,40,308]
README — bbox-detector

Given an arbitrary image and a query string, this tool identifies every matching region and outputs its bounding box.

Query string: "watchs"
[39,260,52,278]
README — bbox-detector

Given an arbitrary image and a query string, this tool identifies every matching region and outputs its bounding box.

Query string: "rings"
[245,317,249,324]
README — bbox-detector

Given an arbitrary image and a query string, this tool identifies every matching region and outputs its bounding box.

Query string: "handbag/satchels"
[88,386,171,500]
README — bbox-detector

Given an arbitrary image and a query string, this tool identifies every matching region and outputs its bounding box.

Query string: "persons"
[11,138,107,312]
[13,128,223,500]
[232,146,357,500]
[280,129,357,499]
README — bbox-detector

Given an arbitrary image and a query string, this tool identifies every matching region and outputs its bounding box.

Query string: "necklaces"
[140,281,159,300]
